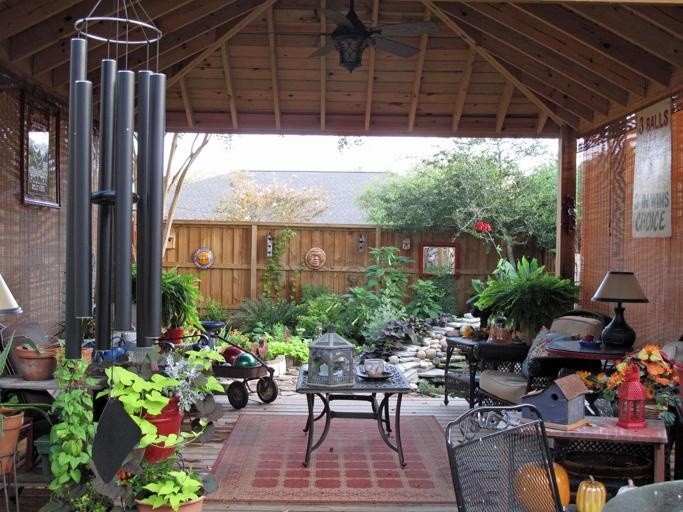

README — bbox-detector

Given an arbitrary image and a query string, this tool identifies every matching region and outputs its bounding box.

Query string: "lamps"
[331,1,371,72]
[591,271,649,349]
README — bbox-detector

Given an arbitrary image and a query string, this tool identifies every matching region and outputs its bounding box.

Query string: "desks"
[600,480,683,512]
[500,416,668,504]
[441,336,527,413]
[546,340,632,370]
[296,365,410,468]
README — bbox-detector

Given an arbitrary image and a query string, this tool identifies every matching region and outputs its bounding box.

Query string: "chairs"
[477,309,611,408]
[445,403,564,512]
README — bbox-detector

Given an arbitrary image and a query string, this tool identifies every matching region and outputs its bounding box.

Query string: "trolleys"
[192,332,276,410]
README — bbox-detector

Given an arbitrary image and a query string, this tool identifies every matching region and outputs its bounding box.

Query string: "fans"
[307,2,440,58]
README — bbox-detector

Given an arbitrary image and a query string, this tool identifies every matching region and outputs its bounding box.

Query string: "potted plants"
[465,256,580,343]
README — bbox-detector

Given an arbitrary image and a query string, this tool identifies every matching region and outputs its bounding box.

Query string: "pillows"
[522,325,580,383]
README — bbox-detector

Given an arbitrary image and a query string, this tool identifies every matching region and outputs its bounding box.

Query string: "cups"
[364,359,384,378]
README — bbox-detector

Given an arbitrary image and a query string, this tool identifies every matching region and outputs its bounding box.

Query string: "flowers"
[577,343,683,425]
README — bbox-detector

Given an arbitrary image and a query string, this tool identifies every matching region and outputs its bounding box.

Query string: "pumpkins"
[516,460,570,512]
[576,475,606,512]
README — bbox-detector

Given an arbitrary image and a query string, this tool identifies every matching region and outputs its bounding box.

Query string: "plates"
[579,340,602,347]
[356,372,393,381]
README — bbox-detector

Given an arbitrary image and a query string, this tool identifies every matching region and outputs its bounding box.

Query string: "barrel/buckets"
[140,397,186,464]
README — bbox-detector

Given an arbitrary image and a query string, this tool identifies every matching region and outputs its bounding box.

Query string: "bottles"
[492,319,509,343]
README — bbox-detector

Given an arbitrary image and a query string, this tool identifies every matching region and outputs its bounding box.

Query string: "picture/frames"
[19,85,62,208]
